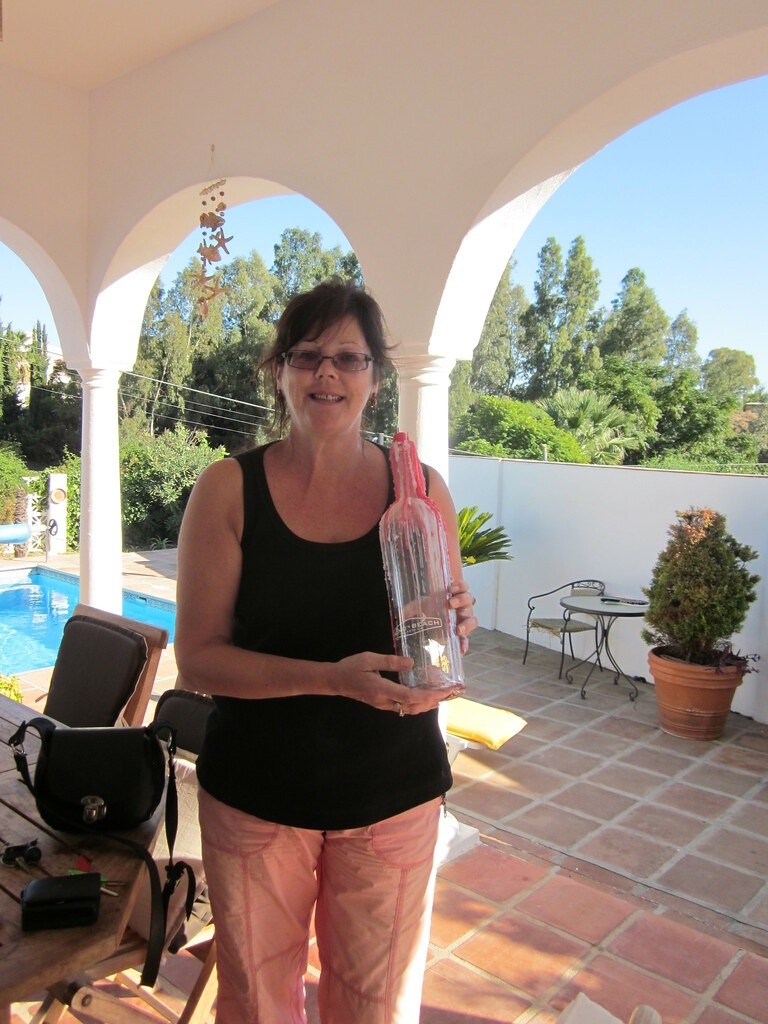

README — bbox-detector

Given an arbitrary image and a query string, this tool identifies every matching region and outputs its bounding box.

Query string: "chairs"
[28,602,169,731]
[522,579,606,680]
[145,669,216,766]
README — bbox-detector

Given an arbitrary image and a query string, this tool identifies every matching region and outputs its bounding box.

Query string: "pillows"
[444,697,527,751]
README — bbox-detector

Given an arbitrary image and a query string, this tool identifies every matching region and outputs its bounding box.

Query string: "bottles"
[377,440,465,700]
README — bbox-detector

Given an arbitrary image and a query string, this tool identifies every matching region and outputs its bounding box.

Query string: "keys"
[2,843,33,874]
[98,886,118,898]
[104,879,127,887]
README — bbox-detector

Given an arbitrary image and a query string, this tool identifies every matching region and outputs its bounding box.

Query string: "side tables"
[560,595,651,701]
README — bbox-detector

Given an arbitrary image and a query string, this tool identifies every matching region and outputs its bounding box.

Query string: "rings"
[395,702,405,718]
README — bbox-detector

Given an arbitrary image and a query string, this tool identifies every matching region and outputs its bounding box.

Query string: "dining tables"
[0,694,220,1024]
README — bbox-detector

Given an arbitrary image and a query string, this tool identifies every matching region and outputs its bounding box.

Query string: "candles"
[379,430,452,642]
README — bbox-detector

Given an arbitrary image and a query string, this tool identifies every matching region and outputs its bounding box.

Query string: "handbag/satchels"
[34,726,164,833]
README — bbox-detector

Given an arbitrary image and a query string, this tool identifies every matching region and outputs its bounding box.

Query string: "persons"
[162,277,479,1023]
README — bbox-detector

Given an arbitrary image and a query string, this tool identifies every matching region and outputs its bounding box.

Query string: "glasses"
[279,348,377,374]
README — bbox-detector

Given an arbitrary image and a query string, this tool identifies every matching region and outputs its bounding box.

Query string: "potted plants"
[640,505,761,742]
